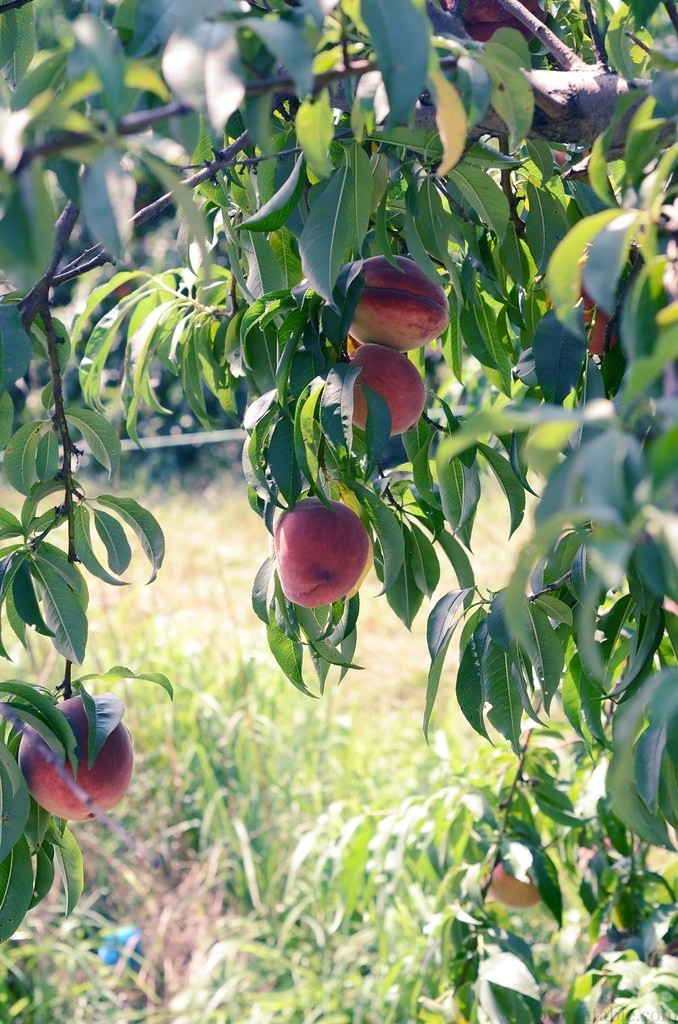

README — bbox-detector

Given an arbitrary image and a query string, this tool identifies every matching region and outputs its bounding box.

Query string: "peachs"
[274,0,678,607]
[487,821,678,1024]
[19,698,134,823]
